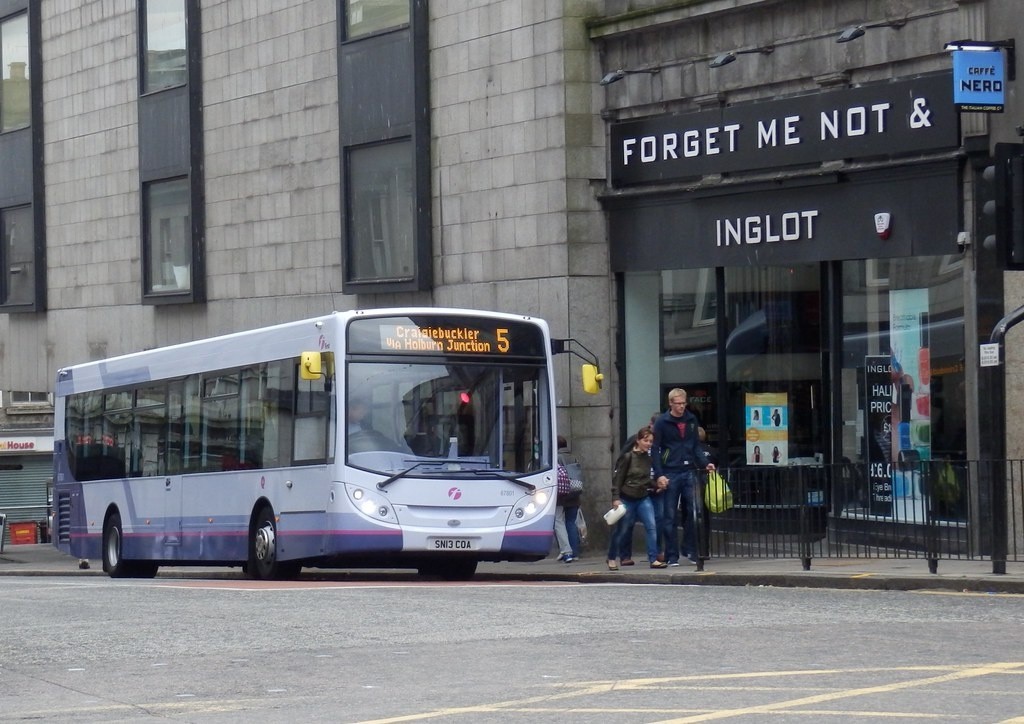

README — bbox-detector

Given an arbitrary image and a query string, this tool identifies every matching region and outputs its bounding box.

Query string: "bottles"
[603,504,627,526]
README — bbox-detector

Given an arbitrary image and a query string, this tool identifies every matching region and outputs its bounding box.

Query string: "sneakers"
[681,550,698,564]
[564,555,573,562]
[666,559,680,566]
[621,558,634,565]
[657,554,665,560]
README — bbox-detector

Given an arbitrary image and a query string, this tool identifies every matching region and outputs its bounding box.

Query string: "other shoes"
[573,554,578,560]
[556,554,563,560]
[606,558,618,570]
[650,560,667,568]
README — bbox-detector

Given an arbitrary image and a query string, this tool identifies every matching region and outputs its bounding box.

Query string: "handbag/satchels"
[705,470,733,513]
[937,462,961,502]
[574,508,587,538]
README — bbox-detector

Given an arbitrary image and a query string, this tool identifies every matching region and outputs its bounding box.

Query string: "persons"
[605,428,667,570]
[651,388,716,566]
[557,435,581,560]
[771,446,782,463]
[753,409,759,420]
[772,409,780,426]
[697,427,712,479]
[348,398,377,451]
[553,506,573,562]
[621,413,666,565]
[753,446,762,463]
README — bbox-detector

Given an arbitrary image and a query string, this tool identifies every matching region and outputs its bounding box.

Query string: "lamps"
[600,67,661,86]
[835,18,908,45]
[707,45,775,69]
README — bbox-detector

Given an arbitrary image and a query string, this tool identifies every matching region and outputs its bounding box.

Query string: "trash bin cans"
[8,520,38,545]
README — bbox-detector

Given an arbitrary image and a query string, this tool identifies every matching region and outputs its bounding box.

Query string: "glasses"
[672,402,687,406]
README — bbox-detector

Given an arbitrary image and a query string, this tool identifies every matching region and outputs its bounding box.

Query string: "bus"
[45,306,604,581]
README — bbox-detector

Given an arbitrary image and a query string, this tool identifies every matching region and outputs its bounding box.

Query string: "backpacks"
[558,464,571,495]
[564,458,583,492]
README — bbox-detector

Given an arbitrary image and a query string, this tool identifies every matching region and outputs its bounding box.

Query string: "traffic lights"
[979,141,1024,271]
